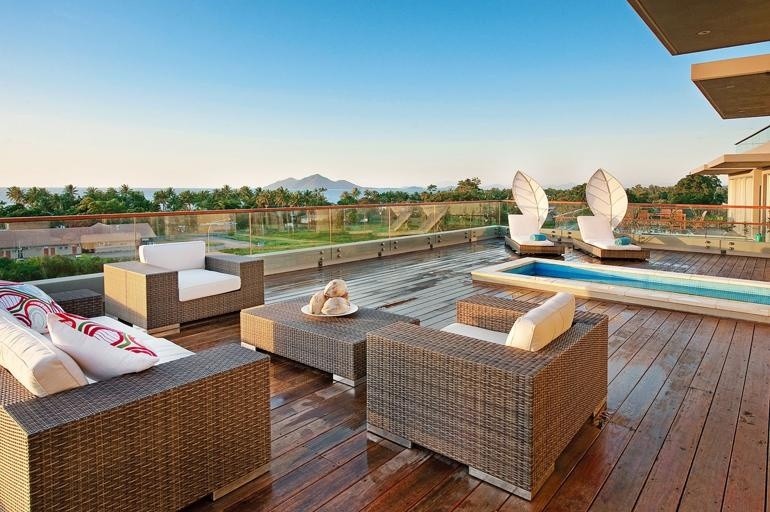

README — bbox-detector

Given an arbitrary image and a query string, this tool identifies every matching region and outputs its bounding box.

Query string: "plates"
[301,301,358,317]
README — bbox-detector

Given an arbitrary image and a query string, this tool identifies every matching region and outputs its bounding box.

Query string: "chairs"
[577,214,643,252]
[632,208,708,234]
[103,241,265,335]
[364,291,610,501]
[507,213,556,247]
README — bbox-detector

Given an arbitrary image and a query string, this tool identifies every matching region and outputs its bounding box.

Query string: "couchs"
[0,289,276,512]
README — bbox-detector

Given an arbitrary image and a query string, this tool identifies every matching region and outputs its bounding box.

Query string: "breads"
[308,278,351,315]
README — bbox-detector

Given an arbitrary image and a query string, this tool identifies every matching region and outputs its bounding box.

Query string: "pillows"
[530,234,546,240]
[0,278,162,383]
[615,236,630,245]
